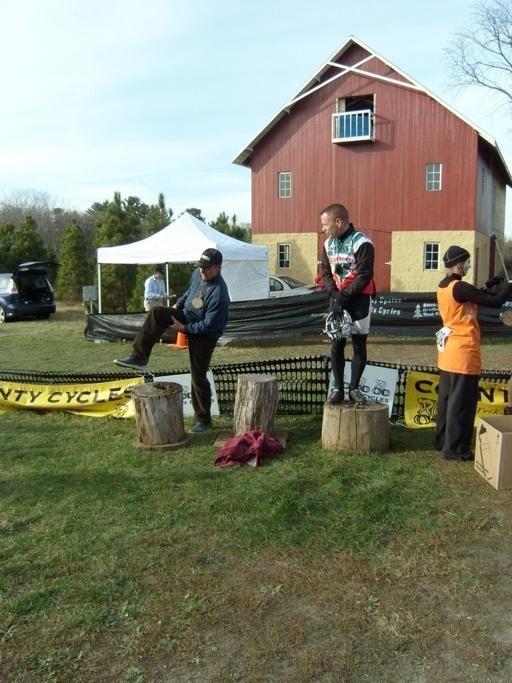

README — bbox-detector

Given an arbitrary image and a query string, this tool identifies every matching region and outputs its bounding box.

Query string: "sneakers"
[436,444,474,460]
[349,387,367,405]
[113,356,147,371]
[326,387,345,404]
[188,419,213,433]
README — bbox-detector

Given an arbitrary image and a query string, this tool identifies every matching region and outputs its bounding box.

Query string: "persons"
[316,204,376,405]
[143,266,169,312]
[435,245,512,461]
[113,249,231,435]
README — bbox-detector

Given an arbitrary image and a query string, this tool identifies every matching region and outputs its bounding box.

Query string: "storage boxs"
[472,412,512,491]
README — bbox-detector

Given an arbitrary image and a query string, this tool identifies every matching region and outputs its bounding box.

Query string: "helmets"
[323,309,353,340]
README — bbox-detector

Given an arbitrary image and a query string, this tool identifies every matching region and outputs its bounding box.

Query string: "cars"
[267,272,321,300]
[0,256,60,325]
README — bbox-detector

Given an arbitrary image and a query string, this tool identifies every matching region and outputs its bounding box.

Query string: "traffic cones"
[170,326,188,350]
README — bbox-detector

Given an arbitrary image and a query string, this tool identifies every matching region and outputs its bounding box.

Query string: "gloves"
[485,275,505,289]
[331,289,346,320]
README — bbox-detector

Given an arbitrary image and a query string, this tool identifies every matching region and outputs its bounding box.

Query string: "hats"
[443,245,470,268]
[193,248,222,269]
[155,266,165,274]
